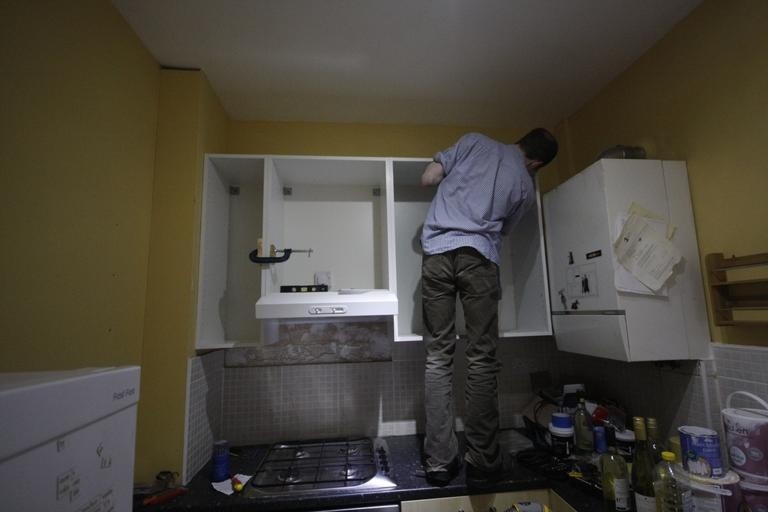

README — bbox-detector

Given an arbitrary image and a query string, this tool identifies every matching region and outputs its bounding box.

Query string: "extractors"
[255,284,398,321]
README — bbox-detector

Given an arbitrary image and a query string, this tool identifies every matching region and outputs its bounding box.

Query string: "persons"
[420,126,559,485]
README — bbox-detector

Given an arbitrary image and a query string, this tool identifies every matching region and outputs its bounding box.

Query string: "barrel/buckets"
[721,390,767,483]
[737,483,768,512]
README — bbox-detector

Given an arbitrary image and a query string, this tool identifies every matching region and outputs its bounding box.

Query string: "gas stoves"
[240,435,398,498]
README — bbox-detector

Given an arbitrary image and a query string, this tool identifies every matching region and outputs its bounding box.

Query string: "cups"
[314,271,331,289]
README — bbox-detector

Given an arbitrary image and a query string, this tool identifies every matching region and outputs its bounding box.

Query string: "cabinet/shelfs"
[191,149,265,348]
[540,157,633,361]
[266,155,390,295]
[391,157,552,343]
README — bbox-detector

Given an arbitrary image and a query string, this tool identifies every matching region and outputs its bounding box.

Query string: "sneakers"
[430,461,491,488]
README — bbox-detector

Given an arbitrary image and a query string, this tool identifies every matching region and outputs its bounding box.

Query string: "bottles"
[213,438,230,482]
[548,389,692,512]
[151,470,176,497]
